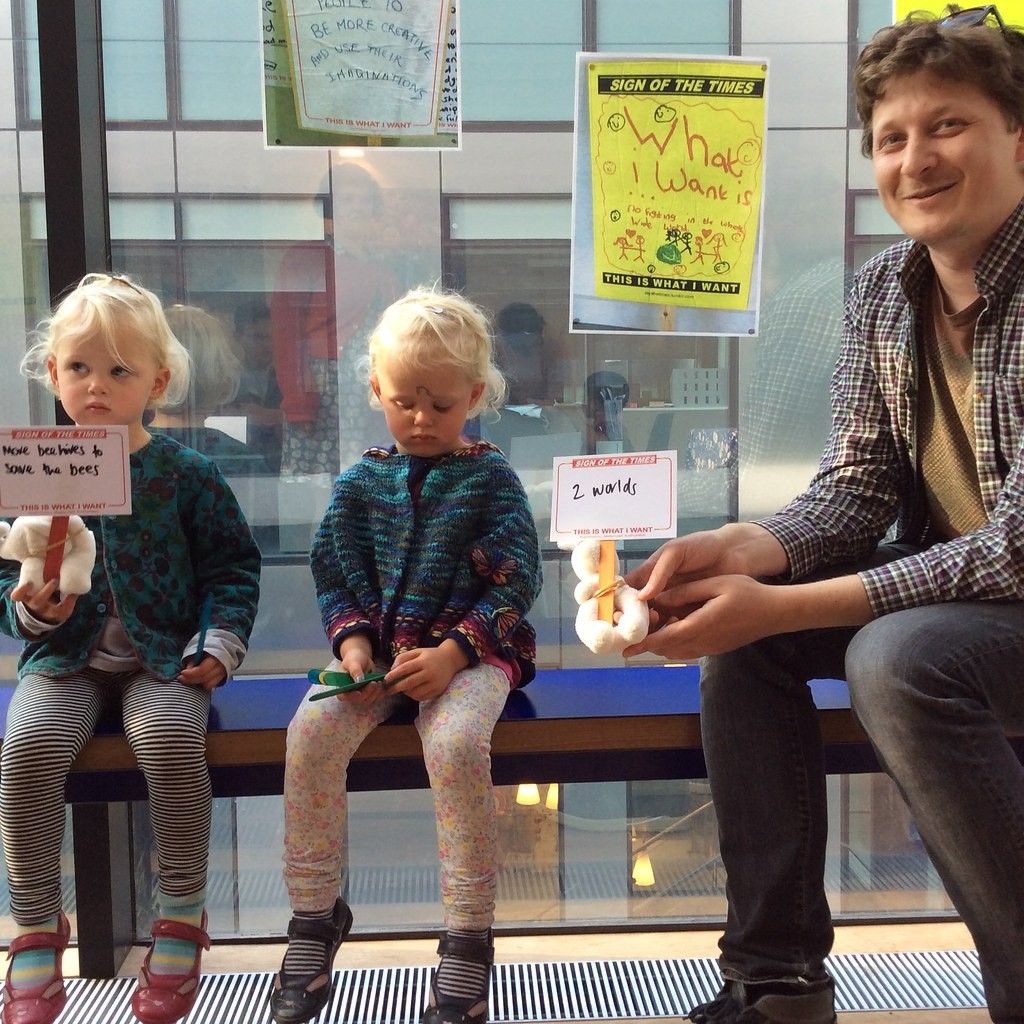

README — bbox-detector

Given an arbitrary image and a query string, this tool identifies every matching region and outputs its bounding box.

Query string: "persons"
[0,273,257,1024]
[612,10,1024,1024]
[492,304,637,455]
[271,282,544,1024]
[274,165,381,476]
[145,304,278,558]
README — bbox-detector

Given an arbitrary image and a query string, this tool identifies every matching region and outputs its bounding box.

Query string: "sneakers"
[682,977,837,1024]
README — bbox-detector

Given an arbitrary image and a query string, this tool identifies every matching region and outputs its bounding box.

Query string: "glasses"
[938,4,1006,31]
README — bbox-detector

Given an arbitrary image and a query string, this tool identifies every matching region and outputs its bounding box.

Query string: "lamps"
[546,783,558,810]
[633,826,656,888]
[516,784,541,806]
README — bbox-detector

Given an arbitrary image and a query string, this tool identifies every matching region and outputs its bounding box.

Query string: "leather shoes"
[130,905,210,1024]
[2,911,71,1024]
[424,928,495,1024]
[270,896,353,1024]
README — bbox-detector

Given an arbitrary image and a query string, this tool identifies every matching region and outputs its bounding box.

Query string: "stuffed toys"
[0,516,95,601]
[558,536,649,654]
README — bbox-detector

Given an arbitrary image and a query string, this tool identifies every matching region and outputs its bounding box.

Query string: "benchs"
[0,667,882,978]
